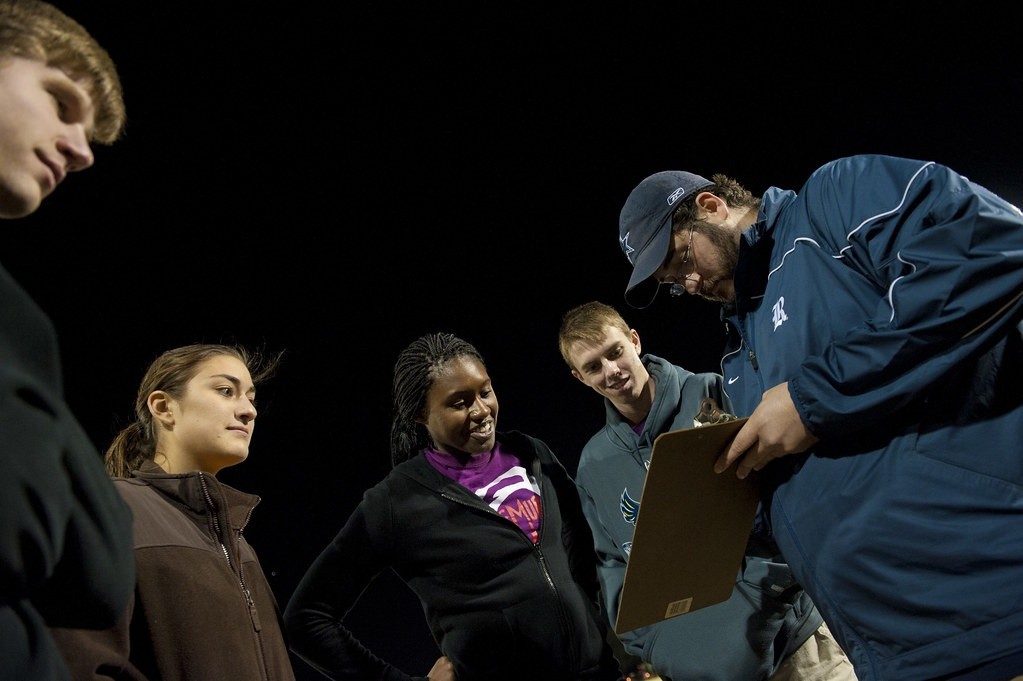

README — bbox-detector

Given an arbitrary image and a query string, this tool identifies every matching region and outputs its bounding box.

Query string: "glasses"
[670,219,695,299]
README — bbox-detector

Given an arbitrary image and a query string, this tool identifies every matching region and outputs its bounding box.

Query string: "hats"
[619,170,716,309]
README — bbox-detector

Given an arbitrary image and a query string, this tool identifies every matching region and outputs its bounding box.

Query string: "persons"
[281,332,635,681]
[0,0,136,681]
[48,344,292,681]
[558,303,823,681]
[617,153,1022,681]
